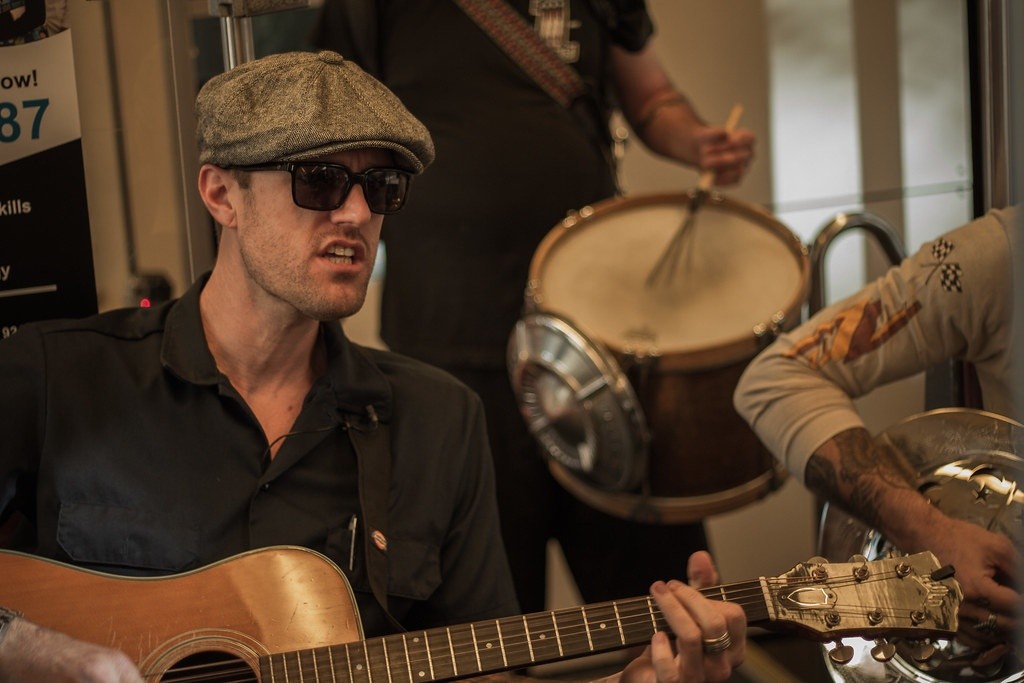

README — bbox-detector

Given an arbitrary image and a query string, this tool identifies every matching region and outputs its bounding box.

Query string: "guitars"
[815,405,1024,683]
[1,545,965,683]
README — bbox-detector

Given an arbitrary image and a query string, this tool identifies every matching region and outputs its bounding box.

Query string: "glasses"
[221,161,415,215]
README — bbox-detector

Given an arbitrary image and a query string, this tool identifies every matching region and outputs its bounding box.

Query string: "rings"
[973,611,997,635]
[702,630,732,654]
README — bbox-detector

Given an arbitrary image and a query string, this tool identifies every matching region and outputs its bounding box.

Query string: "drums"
[506,188,812,530]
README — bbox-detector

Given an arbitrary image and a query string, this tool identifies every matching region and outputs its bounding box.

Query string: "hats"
[194,50,435,176]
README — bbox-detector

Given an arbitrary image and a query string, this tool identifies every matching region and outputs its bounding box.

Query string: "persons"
[734,203,1024,638]
[313,0,756,613]
[0,50,746,683]
